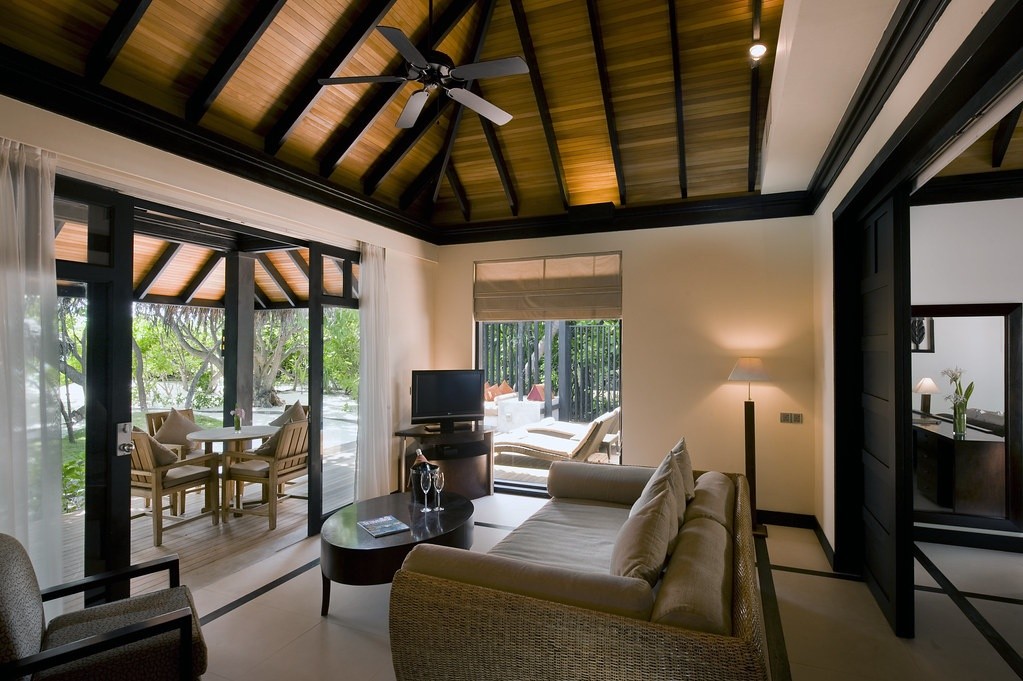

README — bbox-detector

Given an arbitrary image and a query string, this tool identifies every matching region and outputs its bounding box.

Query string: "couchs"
[0,530,209,681]
[389,460,772,681]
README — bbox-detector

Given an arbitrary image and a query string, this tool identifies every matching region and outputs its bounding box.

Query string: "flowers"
[229,407,245,418]
[941,366,974,408]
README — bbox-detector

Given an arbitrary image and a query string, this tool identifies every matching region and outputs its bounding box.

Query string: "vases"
[953,405,968,435]
[234,414,241,432]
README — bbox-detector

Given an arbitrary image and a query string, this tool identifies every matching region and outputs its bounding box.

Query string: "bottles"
[415,449,430,471]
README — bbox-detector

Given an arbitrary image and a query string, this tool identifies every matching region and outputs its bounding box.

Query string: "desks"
[912,421,1006,519]
[320,489,474,616]
[186,423,282,512]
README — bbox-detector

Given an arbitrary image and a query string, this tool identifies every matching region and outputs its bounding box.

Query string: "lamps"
[914,377,939,418]
[727,357,770,537]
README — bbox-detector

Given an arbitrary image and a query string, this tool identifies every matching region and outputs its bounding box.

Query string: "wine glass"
[420,472,432,512]
[433,473,444,512]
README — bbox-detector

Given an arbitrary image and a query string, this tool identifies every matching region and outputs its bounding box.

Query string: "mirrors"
[911,302,1023,532]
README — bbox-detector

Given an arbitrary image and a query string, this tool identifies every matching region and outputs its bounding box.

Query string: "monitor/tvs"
[411,370,484,432]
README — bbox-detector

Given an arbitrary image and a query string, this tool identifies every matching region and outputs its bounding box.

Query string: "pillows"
[152,407,207,456]
[132,425,179,477]
[255,418,294,454]
[268,400,306,427]
[484,380,513,402]
[609,435,696,581]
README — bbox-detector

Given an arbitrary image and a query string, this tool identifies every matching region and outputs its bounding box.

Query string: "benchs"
[492,407,621,463]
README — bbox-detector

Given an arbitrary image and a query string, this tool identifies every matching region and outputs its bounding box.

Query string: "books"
[358,515,410,538]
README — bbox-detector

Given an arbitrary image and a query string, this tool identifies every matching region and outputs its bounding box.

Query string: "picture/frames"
[911,317,935,354]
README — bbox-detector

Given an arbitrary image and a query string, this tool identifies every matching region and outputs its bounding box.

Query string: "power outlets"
[779,412,802,424]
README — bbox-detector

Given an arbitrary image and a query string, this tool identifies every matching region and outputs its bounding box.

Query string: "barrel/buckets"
[410,465,440,508]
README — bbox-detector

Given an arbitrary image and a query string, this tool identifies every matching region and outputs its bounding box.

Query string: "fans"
[316,23,530,130]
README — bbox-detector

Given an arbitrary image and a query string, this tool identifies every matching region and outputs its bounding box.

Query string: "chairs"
[130,404,311,546]
